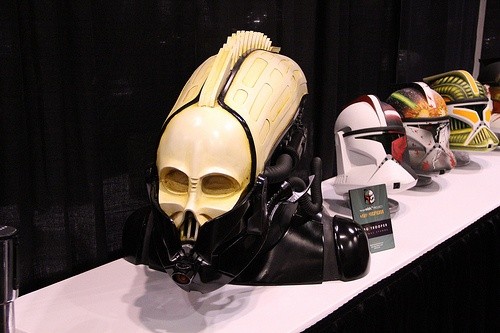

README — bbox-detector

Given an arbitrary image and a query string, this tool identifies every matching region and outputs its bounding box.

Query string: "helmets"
[423,70,499,152]
[334,94,417,194]
[385,82,455,173]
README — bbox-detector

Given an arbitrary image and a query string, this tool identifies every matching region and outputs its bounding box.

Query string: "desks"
[15,144,500,333]
